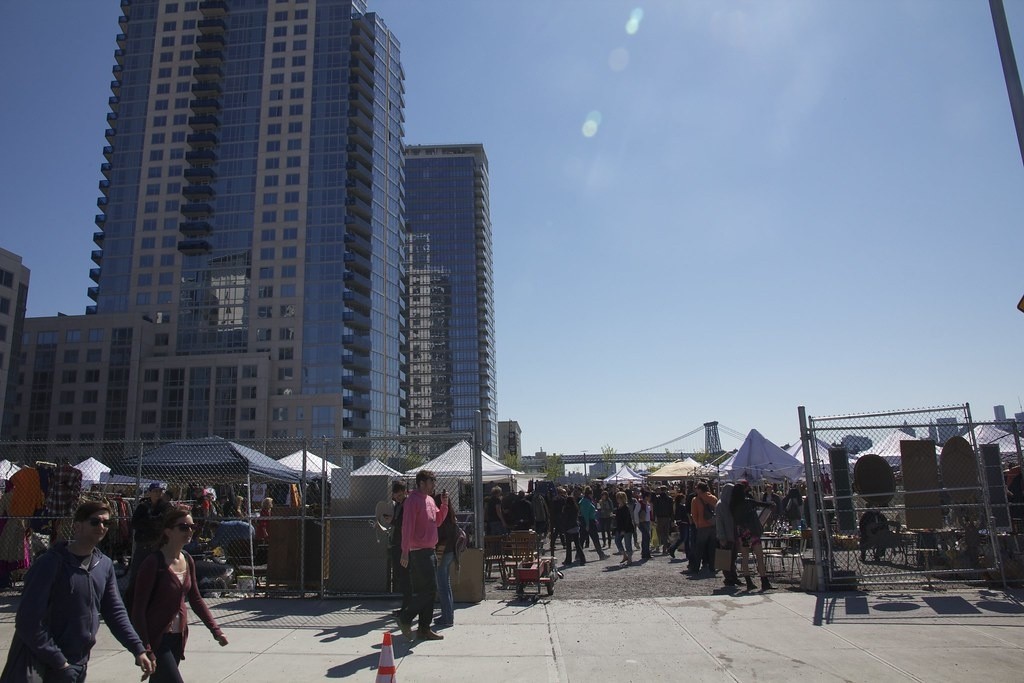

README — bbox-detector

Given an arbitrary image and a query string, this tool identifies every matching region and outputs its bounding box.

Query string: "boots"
[744,575,778,590]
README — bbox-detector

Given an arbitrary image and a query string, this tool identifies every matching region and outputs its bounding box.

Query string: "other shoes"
[858,555,864,561]
[635,543,640,549]
[620,556,632,566]
[434,616,454,627]
[562,561,572,566]
[668,548,675,558]
[563,546,567,549]
[642,555,654,560]
[683,569,699,574]
[609,544,611,548]
[603,544,606,547]
[554,546,556,548]
[600,554,610,560]
[392,609,402,617]
[613,552,624,555]
[585,545,589,548]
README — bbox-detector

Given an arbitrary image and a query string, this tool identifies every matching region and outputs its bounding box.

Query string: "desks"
[761,537,785,571]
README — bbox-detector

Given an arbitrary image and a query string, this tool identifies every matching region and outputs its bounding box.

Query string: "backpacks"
[455,526,468,554]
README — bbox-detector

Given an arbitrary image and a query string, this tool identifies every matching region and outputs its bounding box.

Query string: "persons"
[462,481,807,574]
[859,503,896,561]
[1005,462,1022,516]
[131,480,274,584]
[305,481,321,513]
[714,482,746,588]
[390,470,461,641]
[128,509,229,682]
[15,501,153,683]
[730,484,778,591]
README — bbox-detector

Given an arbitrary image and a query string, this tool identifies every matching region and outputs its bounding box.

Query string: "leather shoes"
[397,619,411,638]
[416,627,444,640]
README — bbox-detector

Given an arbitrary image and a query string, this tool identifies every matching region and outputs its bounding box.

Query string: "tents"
[593,422,1024,502]
[278,438,548,598]
[1,435,328,545]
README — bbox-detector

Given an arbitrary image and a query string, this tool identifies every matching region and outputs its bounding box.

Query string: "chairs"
[484,531,540,584]
[763,535,805,583]
[860,532,938,565]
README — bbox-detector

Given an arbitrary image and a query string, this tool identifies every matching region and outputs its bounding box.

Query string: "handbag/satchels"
[650,524,660,548]
[714,544,732,571]
[704,503,717,520]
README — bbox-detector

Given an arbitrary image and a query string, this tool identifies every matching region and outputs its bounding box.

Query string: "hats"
[695,482,707,491]
[149,483,165,492]
[737,478,751,487]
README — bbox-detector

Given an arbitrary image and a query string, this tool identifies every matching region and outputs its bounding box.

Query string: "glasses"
[172,523,197,532]
[89,517,114,528]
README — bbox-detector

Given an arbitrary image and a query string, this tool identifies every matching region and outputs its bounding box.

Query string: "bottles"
[772,520,789,537]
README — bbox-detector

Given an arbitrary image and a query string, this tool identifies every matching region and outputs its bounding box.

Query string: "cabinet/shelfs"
[265,506,323,598]
